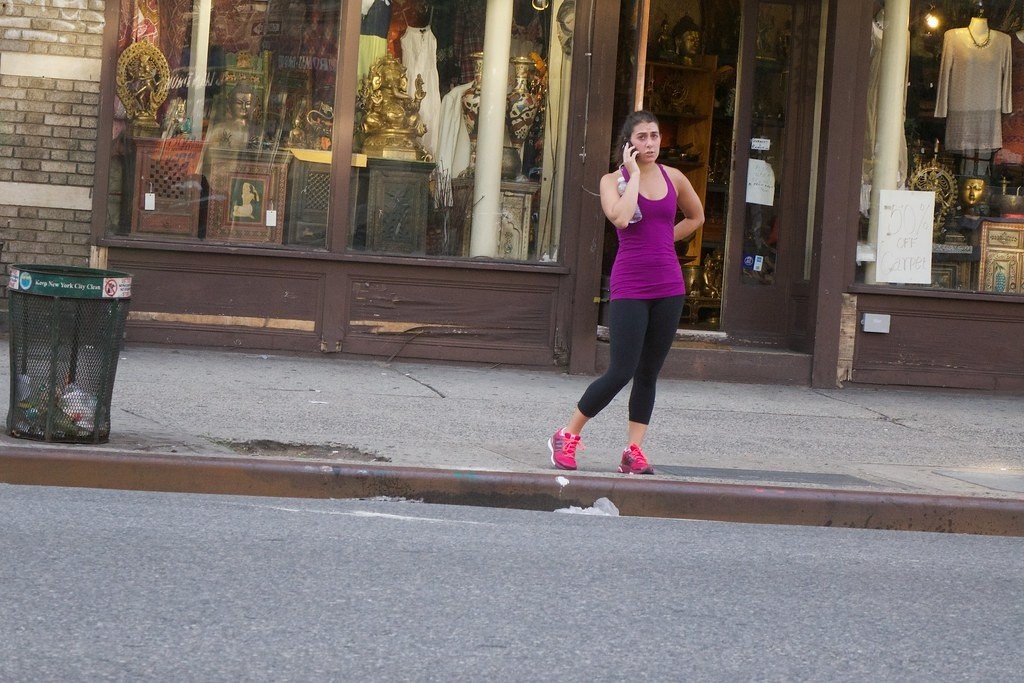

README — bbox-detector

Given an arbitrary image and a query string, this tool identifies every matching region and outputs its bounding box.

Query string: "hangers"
[392,0,442,16]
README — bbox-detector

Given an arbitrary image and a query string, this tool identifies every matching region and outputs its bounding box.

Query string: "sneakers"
[618,444,655,475]
[548,427,585,470]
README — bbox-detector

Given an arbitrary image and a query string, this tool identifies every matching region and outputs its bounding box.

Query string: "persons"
[934,17,1014,151]
[288,118,305,144]
[960,165,988,206]
[225,82,255,126]
[131,64,158,113]
[546,111,705,474]
[859,11,911,217]
[176,102,187,117]
[651,18,671,53]
[702,248,724,299]
[671,13,700,66]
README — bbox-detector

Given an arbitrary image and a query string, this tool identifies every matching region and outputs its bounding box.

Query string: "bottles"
[505,57,540,148]
[460,52,483,140]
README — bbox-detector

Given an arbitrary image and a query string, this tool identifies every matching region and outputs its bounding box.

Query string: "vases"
[505,56,540,181]
[460,52,483,177]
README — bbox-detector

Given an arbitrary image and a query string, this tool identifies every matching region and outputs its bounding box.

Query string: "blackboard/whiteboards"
[875,188,936,284]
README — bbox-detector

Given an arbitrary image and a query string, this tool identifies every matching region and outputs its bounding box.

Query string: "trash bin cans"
[6,263,135,445]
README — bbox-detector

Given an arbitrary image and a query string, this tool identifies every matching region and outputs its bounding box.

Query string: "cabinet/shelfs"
[644,51,786,268]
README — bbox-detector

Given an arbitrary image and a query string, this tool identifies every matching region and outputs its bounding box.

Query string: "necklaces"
[872,16,884,30]
[967,26,991,49]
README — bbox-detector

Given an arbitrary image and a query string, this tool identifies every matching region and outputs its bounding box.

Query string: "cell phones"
[621,138,639,162]
